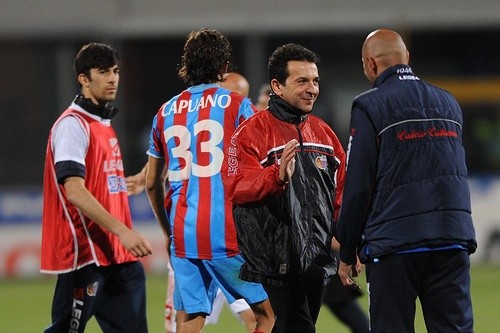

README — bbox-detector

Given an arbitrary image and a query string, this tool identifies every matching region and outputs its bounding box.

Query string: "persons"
[40,43,153,333]
[125,26,369,333]
[339,30,477,333]
